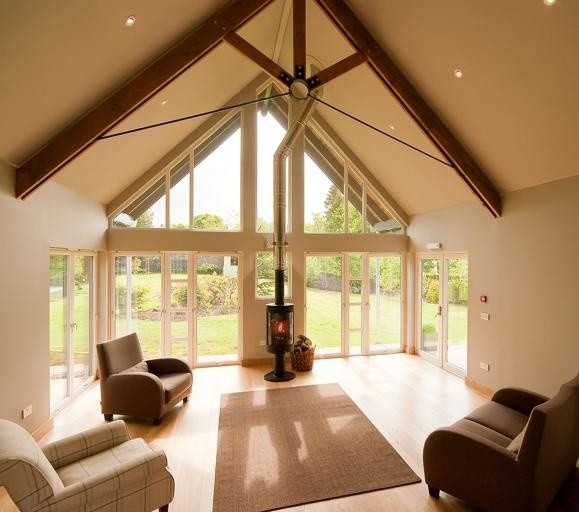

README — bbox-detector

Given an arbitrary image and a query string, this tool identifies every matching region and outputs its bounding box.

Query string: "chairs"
[96,332,194,424]
[423,374,579,512]
[1,420,175,512]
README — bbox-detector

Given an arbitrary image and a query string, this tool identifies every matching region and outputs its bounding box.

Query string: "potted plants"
[288,335,316,371]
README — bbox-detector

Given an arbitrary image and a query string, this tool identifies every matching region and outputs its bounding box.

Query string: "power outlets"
[23,405,33,418]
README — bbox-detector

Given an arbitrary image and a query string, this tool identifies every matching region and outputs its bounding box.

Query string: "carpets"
[213,383,421,512]
